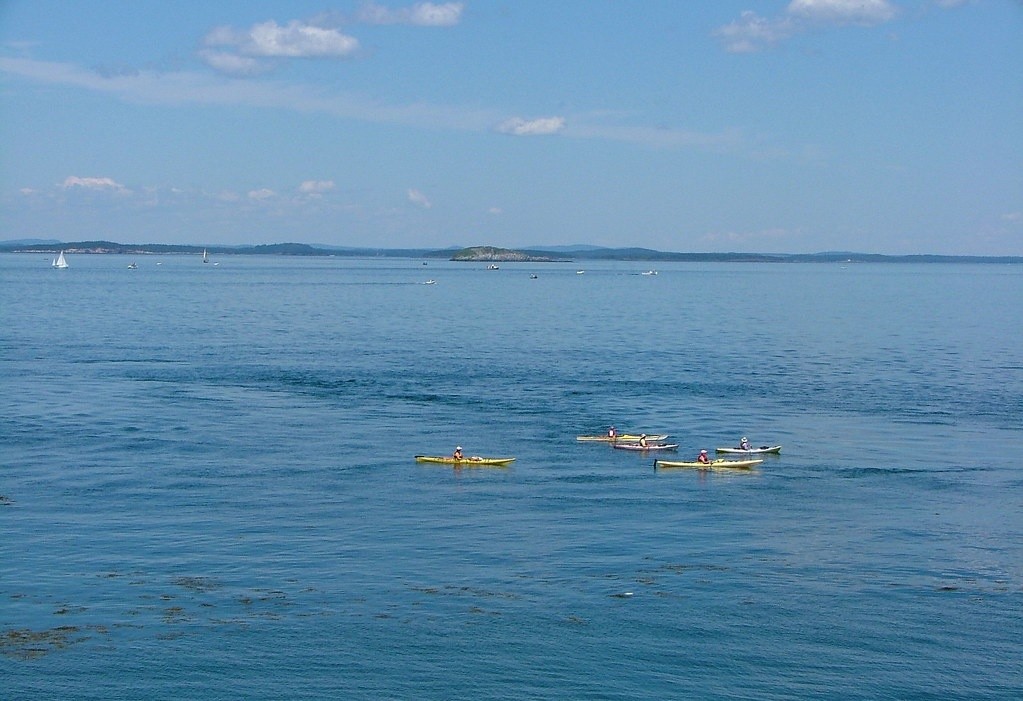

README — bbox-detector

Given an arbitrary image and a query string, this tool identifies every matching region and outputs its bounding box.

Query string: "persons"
[608,426,615,437]
[740,437,750,449]
[698,449,713,464]
[453,446,462,460]
[639,433,648,447]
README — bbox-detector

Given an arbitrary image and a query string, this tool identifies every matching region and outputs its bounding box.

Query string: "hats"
[457,446,463,451]
[701,450,708,454]
[741,437,747,443]
[611,426,614,430]
[641,434,646,438]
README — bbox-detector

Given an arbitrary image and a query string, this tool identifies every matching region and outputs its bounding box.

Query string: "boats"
[642,271,657,275]
[657,459,763,468]
[426,280,437,284]
[615,444,679,451]
[716,445,781,455]
[128,265,139,270]
[417,455,516,466]
[488,265,498,270]
[577,435,668,441]
[576,271,584,274]
[529,273,536,279]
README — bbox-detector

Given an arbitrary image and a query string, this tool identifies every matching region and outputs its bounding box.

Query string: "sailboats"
[202,248,209,264]
[51,250,69,269]
